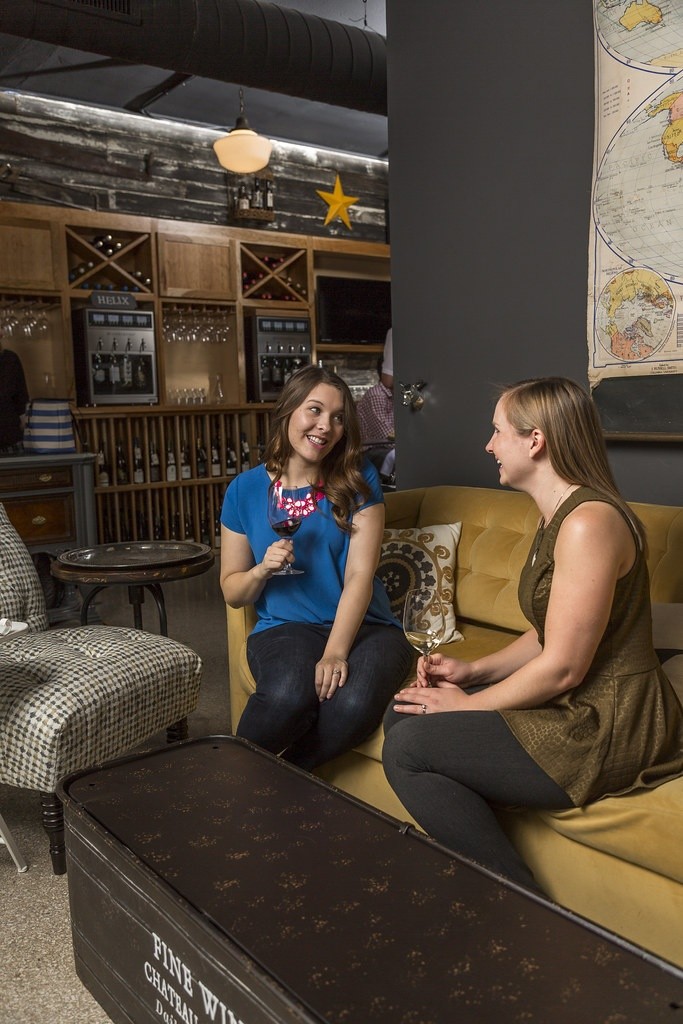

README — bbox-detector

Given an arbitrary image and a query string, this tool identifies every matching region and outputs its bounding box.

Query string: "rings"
[421,704,426,713]
[333,669,340,674]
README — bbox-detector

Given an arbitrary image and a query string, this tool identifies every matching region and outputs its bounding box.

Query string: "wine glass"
[0,305,51,339]
[161,304,231,344]
[268,486,304,575]
[403,589,446,687]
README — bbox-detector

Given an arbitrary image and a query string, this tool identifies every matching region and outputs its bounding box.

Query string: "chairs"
[0,500,204,874]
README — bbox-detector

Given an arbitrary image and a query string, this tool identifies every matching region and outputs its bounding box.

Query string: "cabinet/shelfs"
[1,199,394,559]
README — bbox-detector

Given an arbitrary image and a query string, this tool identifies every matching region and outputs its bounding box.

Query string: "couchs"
[226,485,683,969]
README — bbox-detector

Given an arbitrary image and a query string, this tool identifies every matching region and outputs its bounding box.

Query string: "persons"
[354,327,395,484]
[220,365,414,770]
[380,379,683,897]
[0,326,30,455]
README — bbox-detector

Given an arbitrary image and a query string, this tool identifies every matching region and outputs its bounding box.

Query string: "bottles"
[226,440,236,476]
[114,438,128,485]
[261,345,305,393]
[167,388,206,405]
[238,181,250,210]
[150,441,161,482]
[98,440,109,487]
[212,373,225,405]
[251,172,264,210]
[264,178,273,211]
[133,437,145,484]
[180,446,192,480]
[166,449,177,481]
[242,256,306,300]
[196,438,208,478]
[240,432,250,473]
[92,337,151,394]
[69,234,150,292]
[212,440,222,476]
[106,507,222,549]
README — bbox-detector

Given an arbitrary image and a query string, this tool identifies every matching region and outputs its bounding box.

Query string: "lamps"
[212,85,273,175]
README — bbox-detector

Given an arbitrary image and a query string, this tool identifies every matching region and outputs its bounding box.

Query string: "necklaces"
[531,482,572,567]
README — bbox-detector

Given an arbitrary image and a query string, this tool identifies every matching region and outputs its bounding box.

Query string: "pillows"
[373,521,466,646]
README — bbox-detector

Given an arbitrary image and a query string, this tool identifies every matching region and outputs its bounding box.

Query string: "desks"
[49,538,214,637]
[54,738,683,1023]
